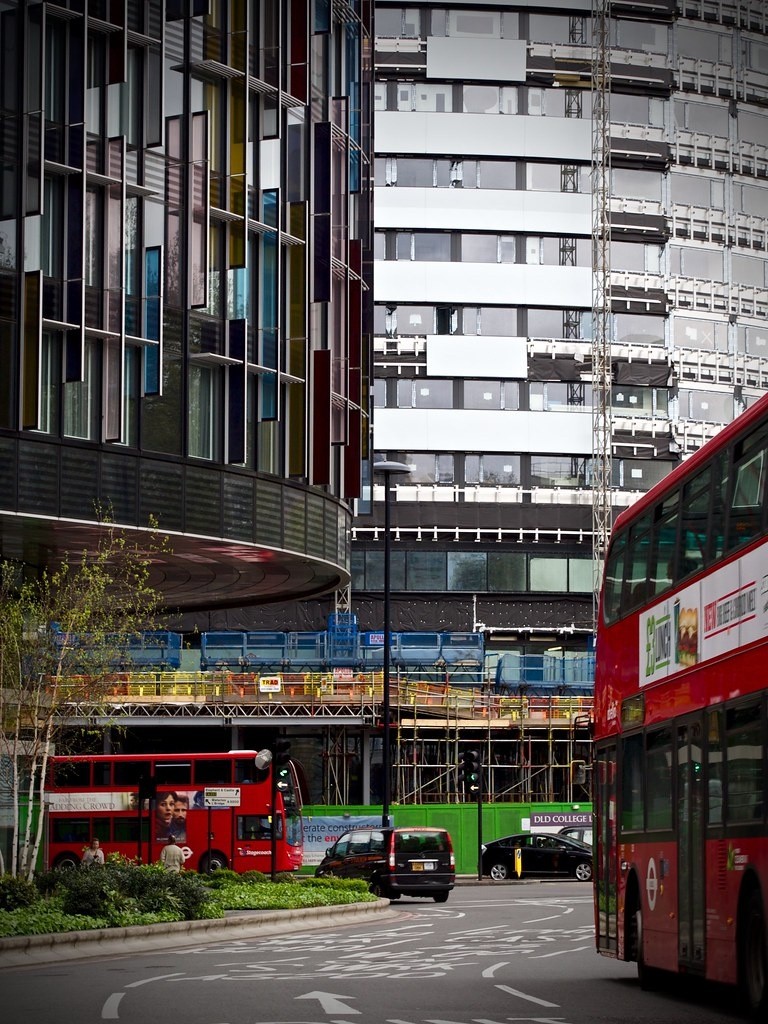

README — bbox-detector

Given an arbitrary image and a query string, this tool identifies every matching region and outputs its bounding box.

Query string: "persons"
[82,838,105,866]
[665,564,674,579]
[192,791,206,809]
[542,839,549,845]
[161,835,185,873]
[155,791,189,841]
[129,793,141,810]
[254,819,270,840]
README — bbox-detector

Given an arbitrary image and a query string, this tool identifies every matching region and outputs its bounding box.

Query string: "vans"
[314,828,455,903]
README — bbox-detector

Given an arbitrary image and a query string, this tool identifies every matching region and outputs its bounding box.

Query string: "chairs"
[423,837,439,850]
[406,836,420,853]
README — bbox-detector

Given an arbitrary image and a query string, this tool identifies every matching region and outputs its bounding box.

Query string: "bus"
[0,754,303,878]
[589,394,768,1024]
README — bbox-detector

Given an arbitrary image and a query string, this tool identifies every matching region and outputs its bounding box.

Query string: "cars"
[558,826,593,845]
[481,833,593,882]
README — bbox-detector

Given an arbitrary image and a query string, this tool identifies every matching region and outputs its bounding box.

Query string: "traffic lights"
[274,739,292,793]
[464,749,482,795]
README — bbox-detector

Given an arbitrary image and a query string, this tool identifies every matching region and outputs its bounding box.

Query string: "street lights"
[371,461,416,829]
